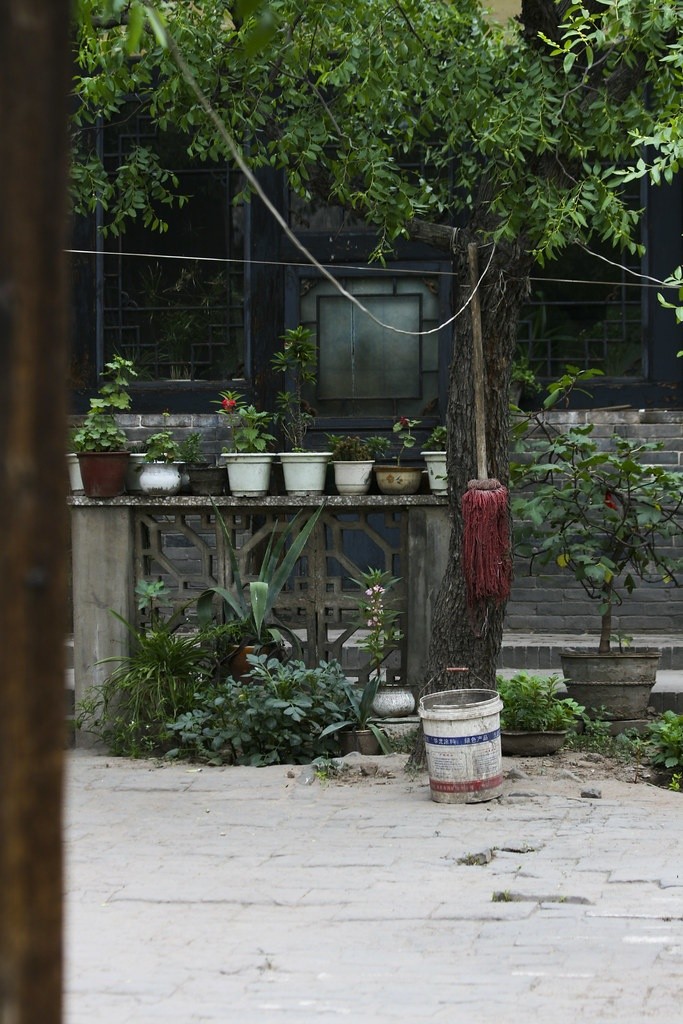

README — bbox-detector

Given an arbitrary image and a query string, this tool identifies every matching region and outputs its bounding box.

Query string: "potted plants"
[326,431,376,495]
[196,492,324,687]
[419,426,449,491]
[77,351,136,496]
[510,423,683,720]
[373,416,428,495]
[492,673,585,756]
[139,411,185,498]
[269,323,334,495]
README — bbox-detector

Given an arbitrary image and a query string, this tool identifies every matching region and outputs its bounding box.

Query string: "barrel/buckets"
[418,668,503,803]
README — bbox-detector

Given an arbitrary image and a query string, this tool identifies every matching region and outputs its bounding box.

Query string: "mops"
[459,245,512,605]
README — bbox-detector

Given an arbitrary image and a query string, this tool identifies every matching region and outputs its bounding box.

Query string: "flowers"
[342,568,406,686]
[71,629,215,743]
[211,389,277,452]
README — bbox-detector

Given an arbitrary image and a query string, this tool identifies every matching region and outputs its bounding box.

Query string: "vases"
[372,688,415,718]
[221,453,278,496]
